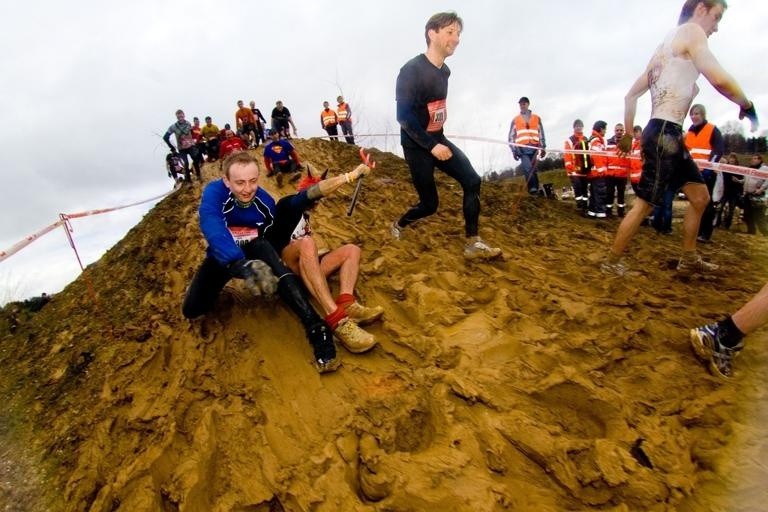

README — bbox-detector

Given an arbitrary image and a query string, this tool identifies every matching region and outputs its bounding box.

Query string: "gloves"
[346,163,371,184]
[617,133,633,153]
[740,100,759,124]
[229,257,279,301]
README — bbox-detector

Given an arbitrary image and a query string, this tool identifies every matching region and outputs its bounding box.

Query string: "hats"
[518,97,530,104]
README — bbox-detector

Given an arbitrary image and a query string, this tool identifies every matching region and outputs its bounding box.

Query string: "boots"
[640,216,654,226]
[713,220,757,234]
[574,196,628,218]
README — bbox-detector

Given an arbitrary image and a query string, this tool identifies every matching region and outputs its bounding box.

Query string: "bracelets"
[348,172,354,184]
[345,172,351,184]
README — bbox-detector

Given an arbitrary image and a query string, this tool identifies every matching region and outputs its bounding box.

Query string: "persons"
[561,102,768,244]
[596,1,760,279]
[265,163,385,355]
[333,95,354,144]
[321,101,338,143]
[687,281,768,382]
[162,99,306,191]
[182,152,341,372]
[507,96,546,195]
[386,11,502,260]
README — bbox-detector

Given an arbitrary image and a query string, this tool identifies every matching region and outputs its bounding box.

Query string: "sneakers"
[305,318,339,373]
[388,222,403,245]
[696,233,708,243]
[329,313,380,354]
[598,260,638,282]
[529,188,538,193]
[689,323,748,384]
[676,257,720,277]
[198,177,206,184]
[336,301,384,326]
[288,172,302,185]
[281,134,292,141]
[200,291,234,341]
[276,173,285,188]
[187,182,194,190]
[463,244,503,261]
